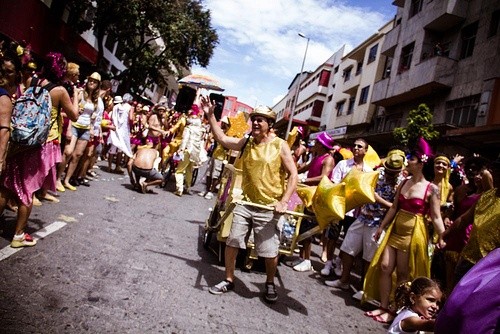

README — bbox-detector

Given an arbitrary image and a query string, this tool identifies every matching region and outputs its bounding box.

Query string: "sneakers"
[209,280,234,294]
[264,282,277,300]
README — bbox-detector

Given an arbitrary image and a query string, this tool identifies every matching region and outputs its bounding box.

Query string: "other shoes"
[10,234,36,247]
[32,169,96,205]
[205,192,214,200]
[198,191,206,196]
[173,191,182,196]
[139,182,146,193]
[294,251,393,323]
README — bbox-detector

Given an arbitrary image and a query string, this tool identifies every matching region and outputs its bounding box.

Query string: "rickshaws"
[200,153,308,274]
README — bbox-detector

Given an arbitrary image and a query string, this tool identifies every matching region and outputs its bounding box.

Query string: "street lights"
[284,32,310,144]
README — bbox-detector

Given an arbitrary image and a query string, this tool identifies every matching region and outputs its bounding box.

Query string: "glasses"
[251,118,268,122]
[353,144,365,149]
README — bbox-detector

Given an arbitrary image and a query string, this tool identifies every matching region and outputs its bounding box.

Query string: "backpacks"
[9,77,58,148]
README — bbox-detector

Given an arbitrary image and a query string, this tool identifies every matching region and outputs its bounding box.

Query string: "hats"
[249,105,276,120]
[317,132,333,149]
[383,154,406,171]
[113,96,123,103]
[87,72,101,84]
[220,116,228,124]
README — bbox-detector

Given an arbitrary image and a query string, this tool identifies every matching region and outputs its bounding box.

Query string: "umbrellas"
[178,72,225,99]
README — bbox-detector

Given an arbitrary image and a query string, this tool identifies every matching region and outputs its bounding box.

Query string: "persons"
[0,40,500,334]
